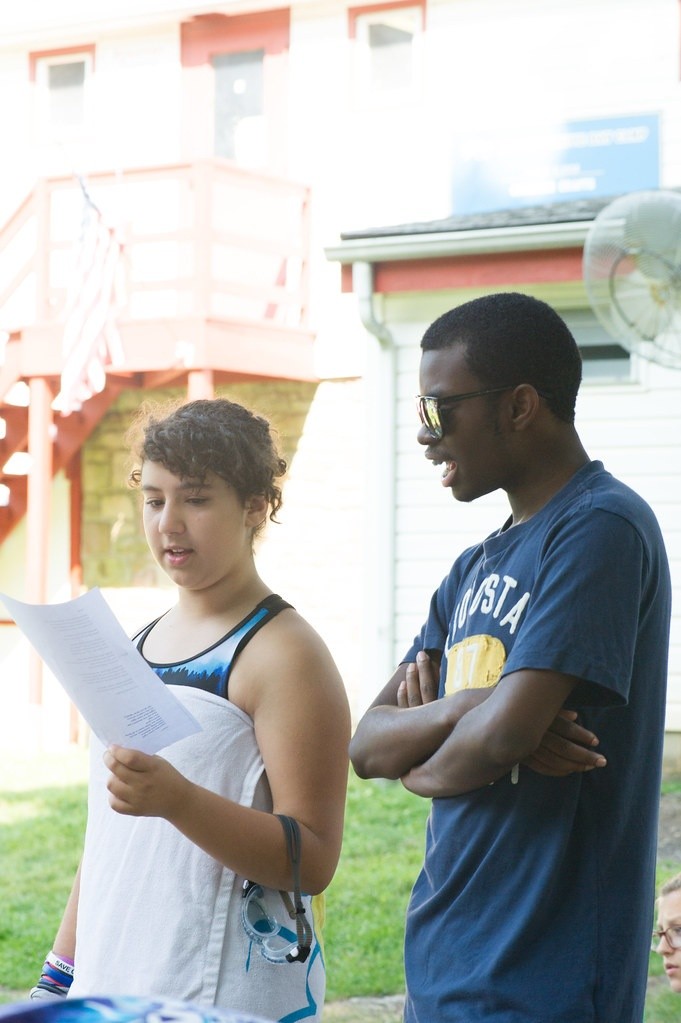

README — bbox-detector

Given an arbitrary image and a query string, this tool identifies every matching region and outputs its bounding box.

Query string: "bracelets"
[28,952,74,999]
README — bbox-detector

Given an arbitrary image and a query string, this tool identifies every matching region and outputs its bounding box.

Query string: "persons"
[649,872,681,995]
[25,397,351,1023]
[348,289,670,1022]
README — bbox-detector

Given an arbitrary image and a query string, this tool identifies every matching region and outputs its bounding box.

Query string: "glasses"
[415,382,556,442]
[239,813,313,965]
